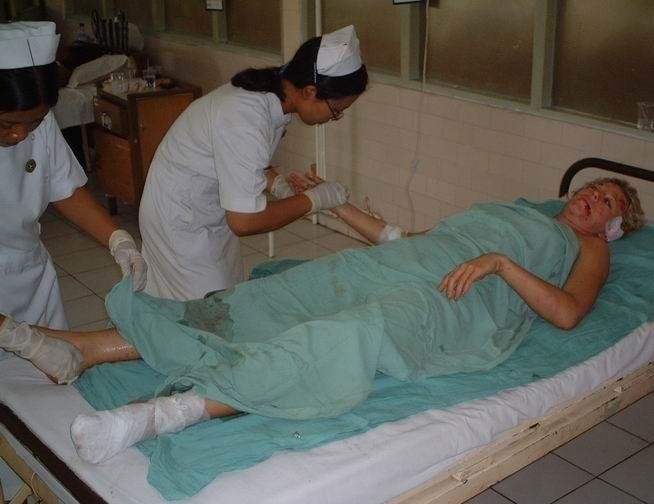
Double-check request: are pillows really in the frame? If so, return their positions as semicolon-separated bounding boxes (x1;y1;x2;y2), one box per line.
65;54;129;88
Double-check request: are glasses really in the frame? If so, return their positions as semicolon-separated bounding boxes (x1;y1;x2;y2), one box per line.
323;97;344;120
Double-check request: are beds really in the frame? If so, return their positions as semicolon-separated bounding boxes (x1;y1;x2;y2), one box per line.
0;155;654;504
51;81;110;174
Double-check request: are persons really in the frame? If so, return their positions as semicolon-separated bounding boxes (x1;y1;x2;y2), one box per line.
0;168;646;466
0;15;147;385
135;20;370;303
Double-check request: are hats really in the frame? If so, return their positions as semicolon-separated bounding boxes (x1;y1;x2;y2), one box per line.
0;18;61;71
314;23;360;80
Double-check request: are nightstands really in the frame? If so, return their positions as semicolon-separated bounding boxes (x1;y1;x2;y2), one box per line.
92;79;203;217
